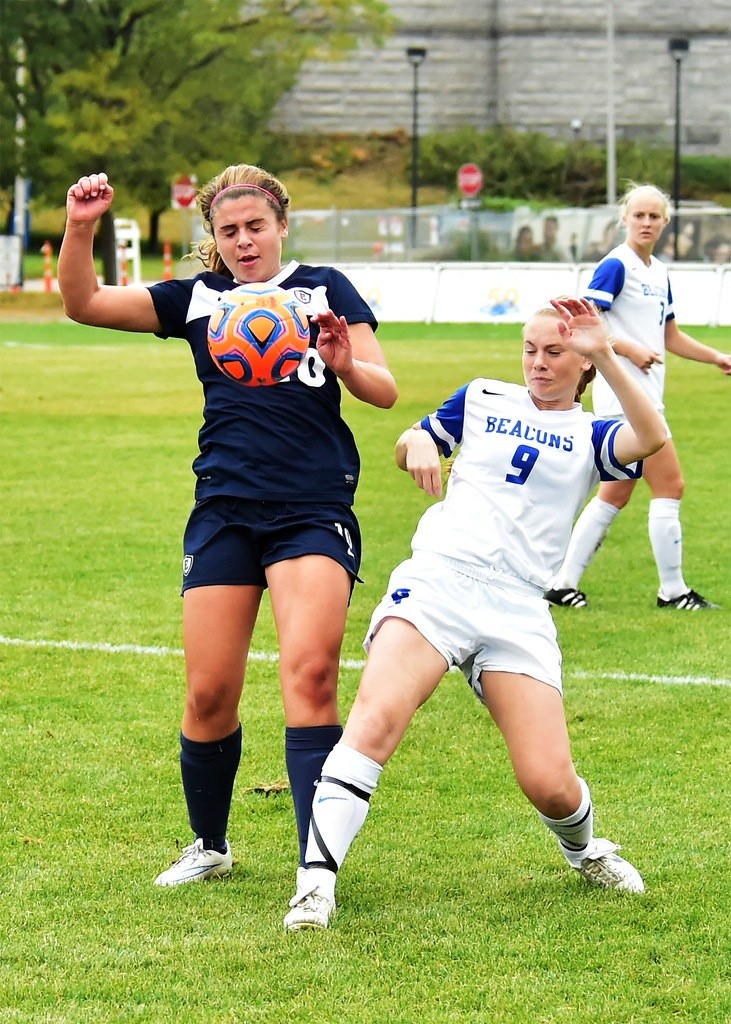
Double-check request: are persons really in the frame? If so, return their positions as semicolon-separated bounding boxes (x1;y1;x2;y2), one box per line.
544;175;731;612
55;162;398;890
283;299;669;936
513;212;731;262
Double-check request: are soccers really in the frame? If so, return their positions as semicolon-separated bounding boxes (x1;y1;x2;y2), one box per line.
208;283;310;386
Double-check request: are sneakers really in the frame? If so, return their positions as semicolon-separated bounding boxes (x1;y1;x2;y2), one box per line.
153;837;232;888
564;836;645;896
544;588;587;611
284;867;336;932
657;589;722;611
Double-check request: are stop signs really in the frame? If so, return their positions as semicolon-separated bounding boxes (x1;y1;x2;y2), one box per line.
172;174;197;204
457;162;484;194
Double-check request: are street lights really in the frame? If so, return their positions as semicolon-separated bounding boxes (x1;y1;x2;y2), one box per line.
405;46;428;251
668;37;690;262
